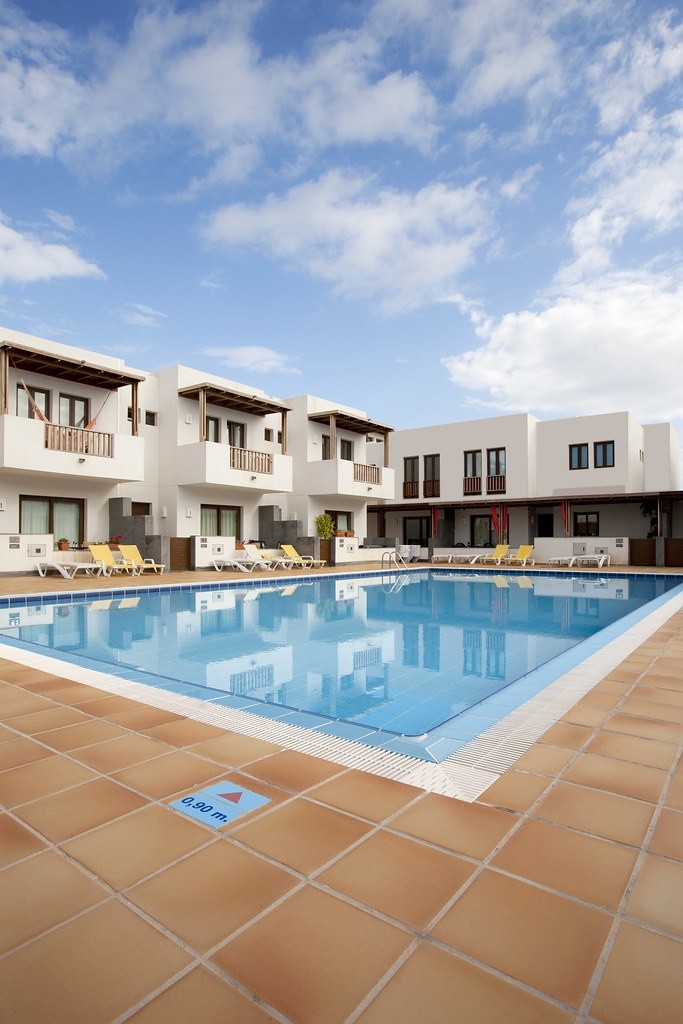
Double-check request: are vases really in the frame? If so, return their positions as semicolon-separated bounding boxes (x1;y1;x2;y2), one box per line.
108;543;122;550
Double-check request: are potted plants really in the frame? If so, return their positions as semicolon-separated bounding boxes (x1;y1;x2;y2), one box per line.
58;538;70;551
337;530;345;537
56;607;70;617
236;541;245;550
345;529;355;537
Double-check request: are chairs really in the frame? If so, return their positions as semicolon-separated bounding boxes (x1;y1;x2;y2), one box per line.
90;545;137;577
117;544;165;575
234;559;272;571
510;576;533;589
37;561;102;581
209;559;251;573
278;584;306;596
281;545;326;569
243;544;273;571
479;545;510;565
453;554;482;564
548;556;578;568
261;552;294;570
486;575;509;589
431;555;455;564
576;554;611;567
506;545;535;566
89;600;113;611
117;598;140;608
244;588;274;602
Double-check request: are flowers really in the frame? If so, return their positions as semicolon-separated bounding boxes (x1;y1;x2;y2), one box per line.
105;534;123;544
